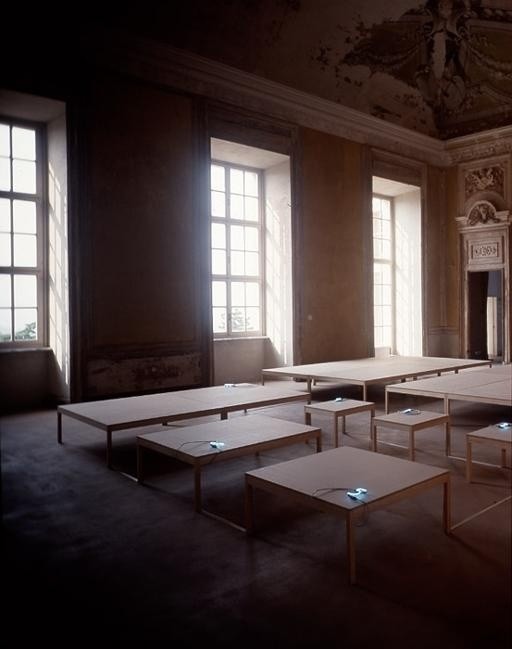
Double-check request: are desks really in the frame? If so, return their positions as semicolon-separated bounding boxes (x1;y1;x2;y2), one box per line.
137;418;321;516
466;423;512;483
262;355;492;401
385;367;511;414
373;411;450;461
305;398;375;446
245;446;450;583
59;386;310;472
